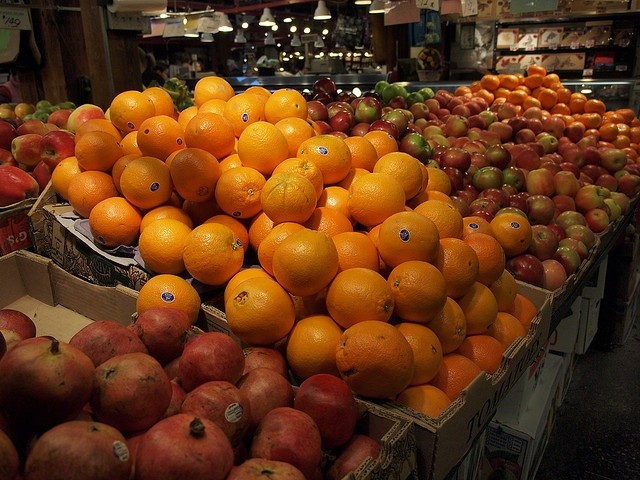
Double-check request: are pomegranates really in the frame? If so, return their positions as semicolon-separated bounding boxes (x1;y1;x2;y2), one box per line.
0;308;378;479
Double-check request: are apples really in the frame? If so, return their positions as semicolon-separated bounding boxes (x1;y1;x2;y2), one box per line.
1;100;106;205
299;79;639;289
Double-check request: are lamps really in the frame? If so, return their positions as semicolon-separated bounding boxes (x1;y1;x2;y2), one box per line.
201;32;214;42
217;14;233;32
242;22;248;28
184;30;199;37
304;25;310;33
322;29;328;34
355;0;372;5
369;0;386;14
259;8;276;26
291;34;300;46
233;28;247;43
272;23;279;31
313;0;331;20
283;16;292;22
263;31;274;45
314;36;325;48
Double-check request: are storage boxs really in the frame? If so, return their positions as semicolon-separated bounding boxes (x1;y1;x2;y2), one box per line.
555;234;600;322
486;353;562;478
1;249;418;480
44;204;553;479
598;296;640;345
603;194;638;249
576;288;604;355
545;297;583;411
0;176;64;259
609;238;640;305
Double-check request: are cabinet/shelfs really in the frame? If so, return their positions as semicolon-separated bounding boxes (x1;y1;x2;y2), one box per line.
493;19;634;77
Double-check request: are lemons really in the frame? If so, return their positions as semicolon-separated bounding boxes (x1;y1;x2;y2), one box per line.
51;77;538;419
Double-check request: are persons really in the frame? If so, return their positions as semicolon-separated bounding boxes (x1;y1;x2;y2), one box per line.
293;58;305;76
157;54;183;65
1;60;36;104
460;48;493;79
194;59;203;72
181;56;193;74
137;46;156;90
226;48;244;72
143;64;170;89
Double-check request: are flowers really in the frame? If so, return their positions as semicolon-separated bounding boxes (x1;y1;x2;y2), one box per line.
418;48;445;69
